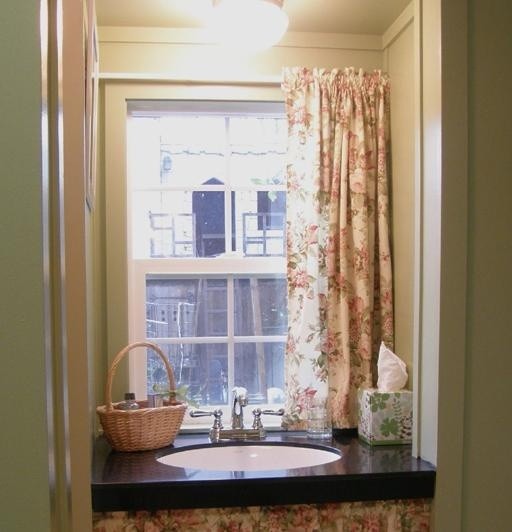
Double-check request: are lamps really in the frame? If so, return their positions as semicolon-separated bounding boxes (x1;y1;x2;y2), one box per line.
212;0;290;49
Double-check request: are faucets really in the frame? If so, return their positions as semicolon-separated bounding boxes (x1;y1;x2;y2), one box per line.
229;384;249;431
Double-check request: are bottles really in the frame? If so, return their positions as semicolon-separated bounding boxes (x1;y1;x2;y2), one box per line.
118;393;140;410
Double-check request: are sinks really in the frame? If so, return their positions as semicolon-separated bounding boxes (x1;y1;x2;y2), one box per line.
154;439;344;474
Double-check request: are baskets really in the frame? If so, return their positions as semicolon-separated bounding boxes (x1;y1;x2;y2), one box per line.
96;342;188;453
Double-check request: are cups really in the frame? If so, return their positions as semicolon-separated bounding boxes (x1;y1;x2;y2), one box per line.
294;395;334;440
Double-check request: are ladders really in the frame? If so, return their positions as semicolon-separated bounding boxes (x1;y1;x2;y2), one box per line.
198;234;243;406
